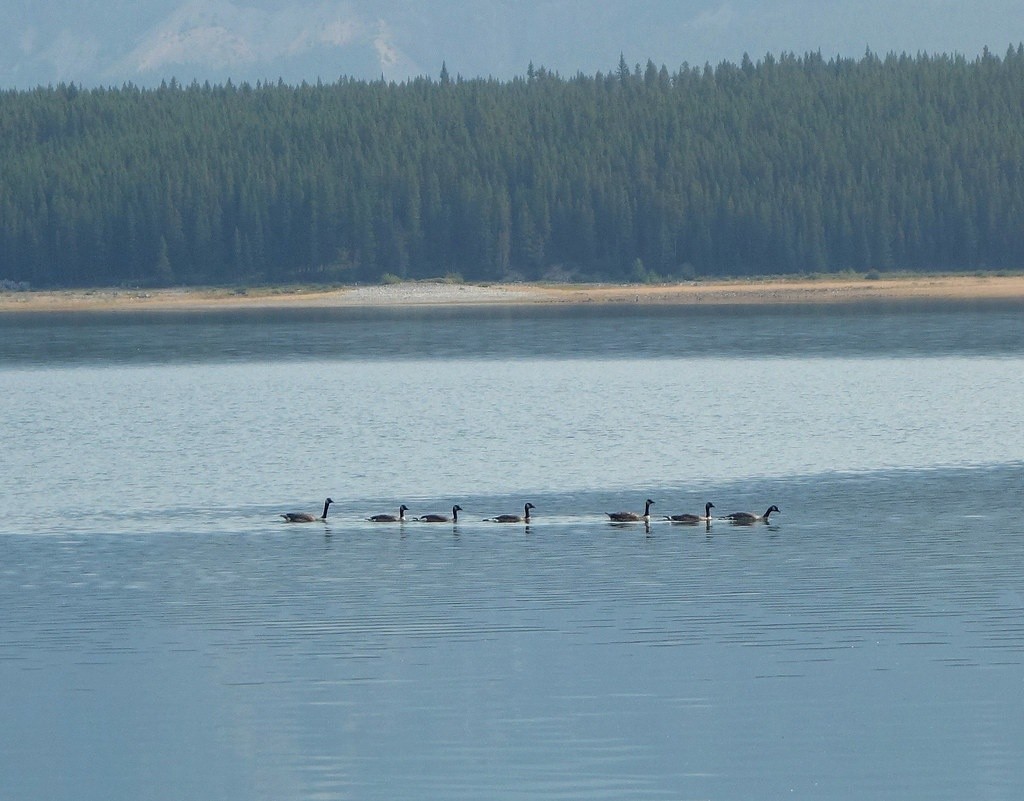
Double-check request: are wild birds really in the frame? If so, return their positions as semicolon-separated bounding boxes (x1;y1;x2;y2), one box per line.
413;505;462;522
482;502;536;523
663;501;716;521
364;505;410;522
605;499;656;522
716;504;780;520
280;498;335;522
